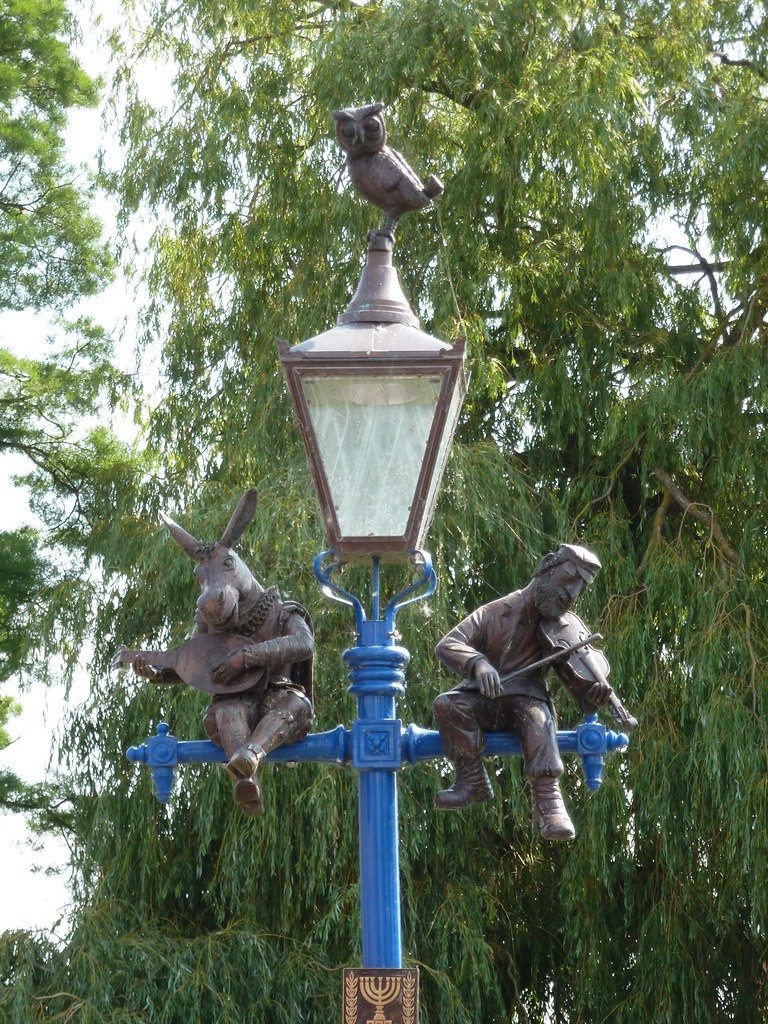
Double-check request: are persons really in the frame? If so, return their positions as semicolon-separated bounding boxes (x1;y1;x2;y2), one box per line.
431;543;614;843
